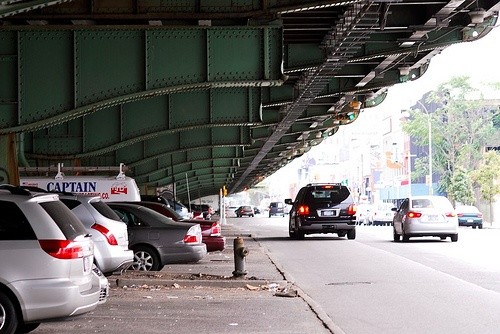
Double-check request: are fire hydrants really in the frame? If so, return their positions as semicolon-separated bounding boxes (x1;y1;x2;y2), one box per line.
232;235;249;279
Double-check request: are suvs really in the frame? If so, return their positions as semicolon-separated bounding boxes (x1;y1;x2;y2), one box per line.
269;182;357;240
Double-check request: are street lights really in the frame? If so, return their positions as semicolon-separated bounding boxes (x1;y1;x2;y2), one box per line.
347;93;435;196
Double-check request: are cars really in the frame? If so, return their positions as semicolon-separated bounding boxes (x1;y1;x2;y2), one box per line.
357;203;396;224
391;195;458;243
103;189;226;274
235;205;262;217
454;205;482;229
0;184;135;334
